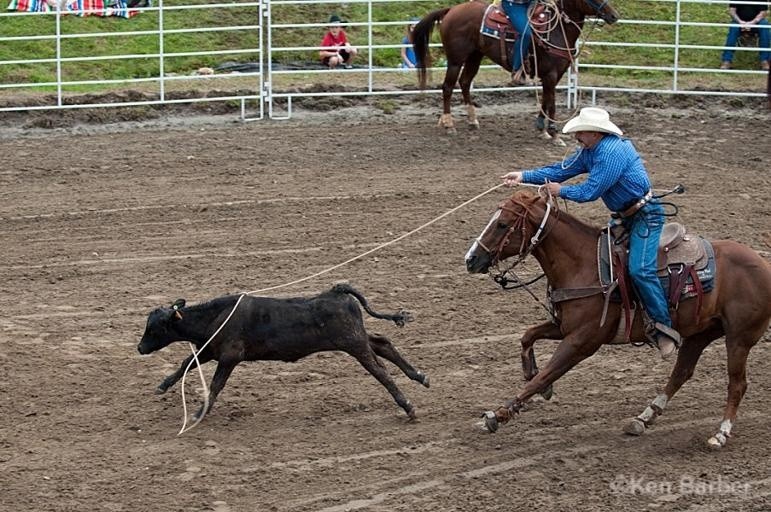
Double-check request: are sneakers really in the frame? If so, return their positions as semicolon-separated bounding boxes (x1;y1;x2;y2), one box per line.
340;62;353;69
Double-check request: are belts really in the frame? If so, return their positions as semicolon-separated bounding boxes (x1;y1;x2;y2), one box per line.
619;188;654;221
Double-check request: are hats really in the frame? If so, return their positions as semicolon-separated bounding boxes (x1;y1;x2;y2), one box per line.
561;106;625;138
321;16;348;28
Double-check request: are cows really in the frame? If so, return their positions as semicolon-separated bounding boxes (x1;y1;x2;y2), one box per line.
136;281;431;423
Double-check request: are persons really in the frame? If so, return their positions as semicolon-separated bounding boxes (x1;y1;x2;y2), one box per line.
718;1;771;71
317;14;354;70
401;17;423;70
502;103;679;358
499;1;542;87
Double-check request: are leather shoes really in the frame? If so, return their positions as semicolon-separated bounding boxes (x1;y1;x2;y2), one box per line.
718;60;735;70
657;335;677;360
511;74;528;86
760;60;771;71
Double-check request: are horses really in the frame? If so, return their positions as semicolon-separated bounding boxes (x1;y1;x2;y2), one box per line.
408;1;621;148
464;189;771;452
